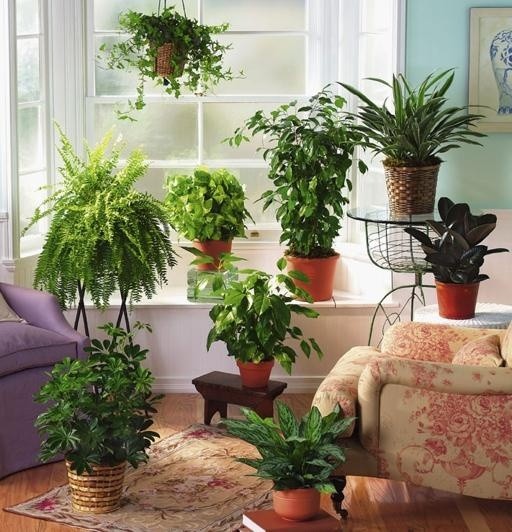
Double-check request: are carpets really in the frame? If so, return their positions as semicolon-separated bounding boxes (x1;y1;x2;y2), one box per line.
3;424;285;532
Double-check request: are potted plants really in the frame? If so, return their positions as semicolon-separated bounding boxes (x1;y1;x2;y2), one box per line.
218;401;357;523
33;321;164;511
223;83;369;303
177;242;324;389
162;169;256;273
402;196;509;319
336;66;498;215
92;5;247;123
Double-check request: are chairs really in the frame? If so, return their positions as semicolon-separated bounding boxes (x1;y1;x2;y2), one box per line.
0;282;93;480
305;313;511;518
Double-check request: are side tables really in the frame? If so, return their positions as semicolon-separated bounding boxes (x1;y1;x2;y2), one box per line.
413;303;512;329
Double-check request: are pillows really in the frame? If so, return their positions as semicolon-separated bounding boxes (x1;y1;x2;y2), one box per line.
0;290;29;324
450;334;505;368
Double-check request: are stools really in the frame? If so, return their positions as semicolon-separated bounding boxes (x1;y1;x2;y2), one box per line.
192;371;286;424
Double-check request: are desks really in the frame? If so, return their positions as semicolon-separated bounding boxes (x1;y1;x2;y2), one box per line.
346;204;482;358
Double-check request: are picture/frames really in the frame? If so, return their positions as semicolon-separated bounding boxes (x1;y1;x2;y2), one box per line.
468;7;512;133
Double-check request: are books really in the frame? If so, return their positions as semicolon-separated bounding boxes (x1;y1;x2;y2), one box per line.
242;505;340;532
